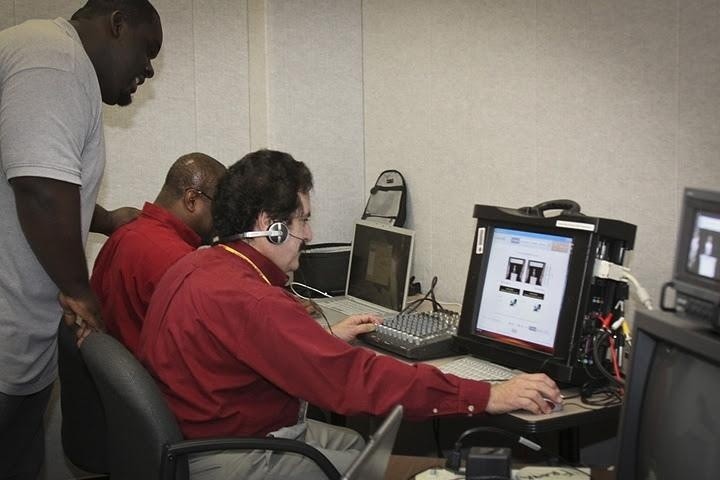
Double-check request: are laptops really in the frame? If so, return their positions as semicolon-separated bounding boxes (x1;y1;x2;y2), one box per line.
313;218;416;332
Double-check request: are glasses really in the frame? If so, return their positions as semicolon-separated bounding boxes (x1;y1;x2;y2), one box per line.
186;188;213;202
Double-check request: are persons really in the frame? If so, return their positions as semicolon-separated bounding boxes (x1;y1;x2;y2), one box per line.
525;268;541;287
0;0;164;480
90;152;322;361
506;264;518;281
136;148;563;480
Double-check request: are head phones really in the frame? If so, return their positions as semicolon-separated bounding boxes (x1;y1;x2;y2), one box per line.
212;221;289;246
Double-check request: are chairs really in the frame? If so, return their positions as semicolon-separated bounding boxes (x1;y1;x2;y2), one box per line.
56;315;111;474
79;330;343;480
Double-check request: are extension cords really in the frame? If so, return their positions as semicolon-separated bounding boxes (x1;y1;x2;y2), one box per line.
414;464;591;480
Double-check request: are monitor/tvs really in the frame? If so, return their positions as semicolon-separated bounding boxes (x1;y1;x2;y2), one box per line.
614;306;720;480
454;203;637;385
659;184;720;322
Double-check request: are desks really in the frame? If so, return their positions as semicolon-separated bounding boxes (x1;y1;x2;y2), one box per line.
301;295;624;466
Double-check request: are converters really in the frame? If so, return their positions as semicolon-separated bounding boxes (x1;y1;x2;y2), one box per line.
464;445;512;480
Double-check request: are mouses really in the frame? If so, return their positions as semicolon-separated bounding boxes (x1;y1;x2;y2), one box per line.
539;397;564;413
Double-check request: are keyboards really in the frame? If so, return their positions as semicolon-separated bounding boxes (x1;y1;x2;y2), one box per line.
440;355;524;384
